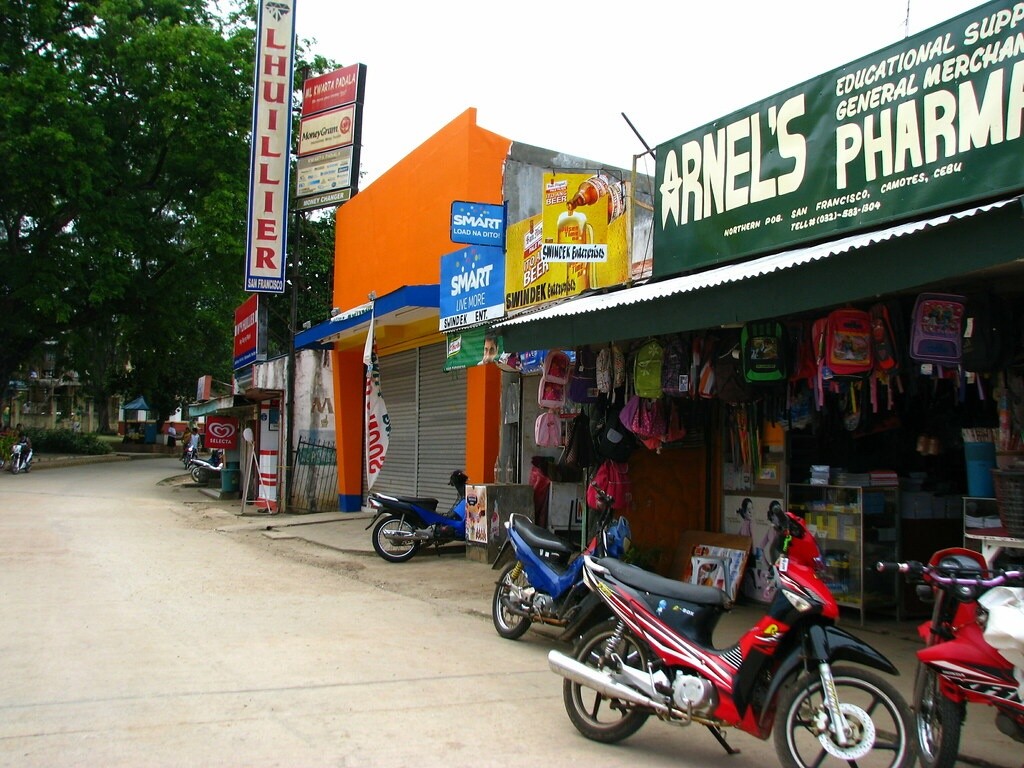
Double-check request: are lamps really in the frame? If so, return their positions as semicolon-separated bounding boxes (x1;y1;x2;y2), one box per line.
368;291;377;300
331;308;340;316
303;321;311;329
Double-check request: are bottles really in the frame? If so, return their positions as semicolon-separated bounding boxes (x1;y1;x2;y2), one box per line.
494;456;502;485
506;456;513;484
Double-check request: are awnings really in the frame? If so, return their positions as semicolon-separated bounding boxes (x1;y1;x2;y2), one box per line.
491;195;1024;355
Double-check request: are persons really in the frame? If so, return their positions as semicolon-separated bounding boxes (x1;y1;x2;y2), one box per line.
167;422;177;454
10;423;31;466
737;498;757;555
761;500;782;570
180;418;201;460
71;410;81;430
478;327;498;365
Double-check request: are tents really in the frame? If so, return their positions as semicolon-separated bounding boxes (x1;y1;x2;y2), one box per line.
123;396;150;422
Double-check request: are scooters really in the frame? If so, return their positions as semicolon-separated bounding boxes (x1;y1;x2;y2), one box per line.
8;443;35;475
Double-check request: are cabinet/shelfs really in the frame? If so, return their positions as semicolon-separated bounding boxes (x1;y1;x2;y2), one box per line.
787;484;898;626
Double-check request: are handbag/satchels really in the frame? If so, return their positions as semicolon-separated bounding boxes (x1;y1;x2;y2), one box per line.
587;459;622;510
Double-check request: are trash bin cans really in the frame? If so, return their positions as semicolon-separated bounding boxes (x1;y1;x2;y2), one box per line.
220;469;240;493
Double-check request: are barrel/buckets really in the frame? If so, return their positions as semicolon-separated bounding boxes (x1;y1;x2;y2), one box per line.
991;469;1024;538
963;442;997;497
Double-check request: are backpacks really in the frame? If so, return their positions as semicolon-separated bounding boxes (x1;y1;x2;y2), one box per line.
556;289;1001;474
538;377;565;409
543;348;570;385
534;410;561;448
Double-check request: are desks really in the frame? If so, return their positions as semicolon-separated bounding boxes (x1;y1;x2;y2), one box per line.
962;533;1023;573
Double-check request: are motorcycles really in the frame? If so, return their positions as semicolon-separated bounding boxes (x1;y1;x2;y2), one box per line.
491;481;638;641
364;469;473;563
179;440;198;471
187;449;225;483
549;503;919;767
874;547;1024;768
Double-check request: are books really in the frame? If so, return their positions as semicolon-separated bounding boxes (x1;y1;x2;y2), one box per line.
833;470;899;487
